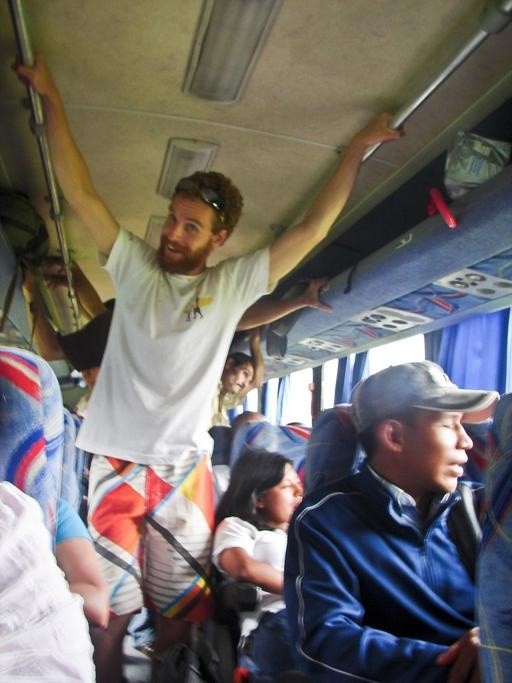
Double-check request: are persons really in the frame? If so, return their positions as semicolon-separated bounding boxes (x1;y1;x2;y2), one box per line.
49;504;112;632
11;49;406;682
278;361;502;682
1;478;102;683
467;393;512;680
23;252;115;372
212;326;267;428
238;275;334;331
210;448;305;674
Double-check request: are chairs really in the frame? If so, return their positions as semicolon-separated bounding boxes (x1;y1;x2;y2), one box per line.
2;346;88;563
200;392;512;683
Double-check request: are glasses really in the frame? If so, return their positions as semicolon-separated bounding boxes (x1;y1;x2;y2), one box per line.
174;178;225;212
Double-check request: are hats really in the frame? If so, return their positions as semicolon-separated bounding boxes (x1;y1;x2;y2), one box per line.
349;362;502;435
